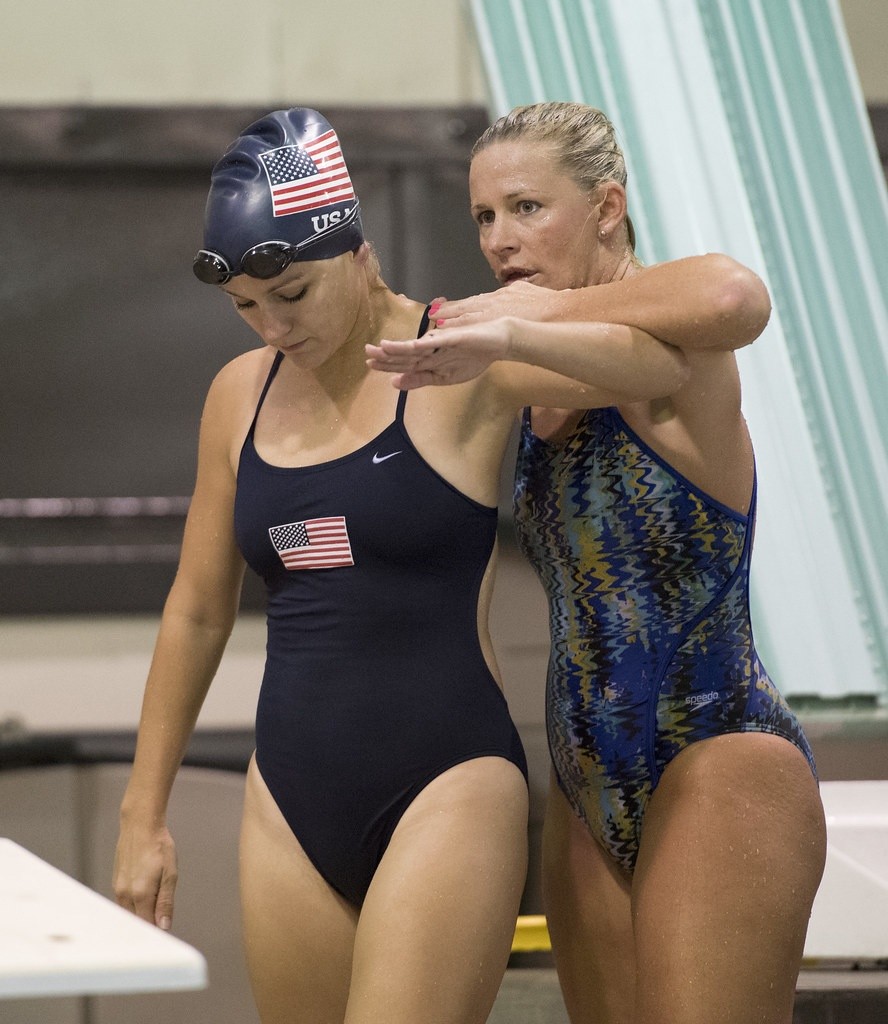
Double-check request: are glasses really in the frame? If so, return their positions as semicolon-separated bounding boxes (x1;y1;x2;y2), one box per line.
192;238;295;285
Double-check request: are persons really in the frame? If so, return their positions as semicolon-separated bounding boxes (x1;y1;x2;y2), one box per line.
114;107;692;1024
431;104;827;1023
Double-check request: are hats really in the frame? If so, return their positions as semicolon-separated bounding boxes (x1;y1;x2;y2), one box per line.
202;107;365;262
